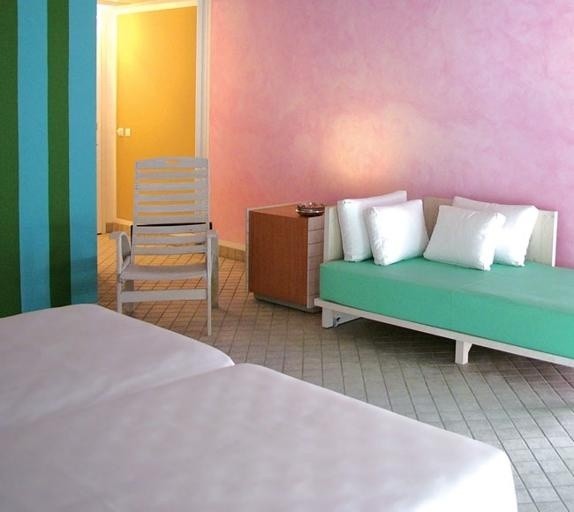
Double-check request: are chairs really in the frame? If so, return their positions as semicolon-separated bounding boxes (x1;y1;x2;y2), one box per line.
115;156;219;336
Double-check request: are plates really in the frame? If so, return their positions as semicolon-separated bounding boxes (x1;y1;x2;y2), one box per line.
297;205;324;216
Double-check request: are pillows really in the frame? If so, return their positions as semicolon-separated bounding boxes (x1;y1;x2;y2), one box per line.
364;199;429;265
423;205;506;272
452;196;539;267
336;190;406;262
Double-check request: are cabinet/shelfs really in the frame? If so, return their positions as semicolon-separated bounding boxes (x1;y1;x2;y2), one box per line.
245;201;325;314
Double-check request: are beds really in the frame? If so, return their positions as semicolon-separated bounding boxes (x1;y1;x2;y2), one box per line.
315;196;574;372
2;361;510;512
0;302;237;427
